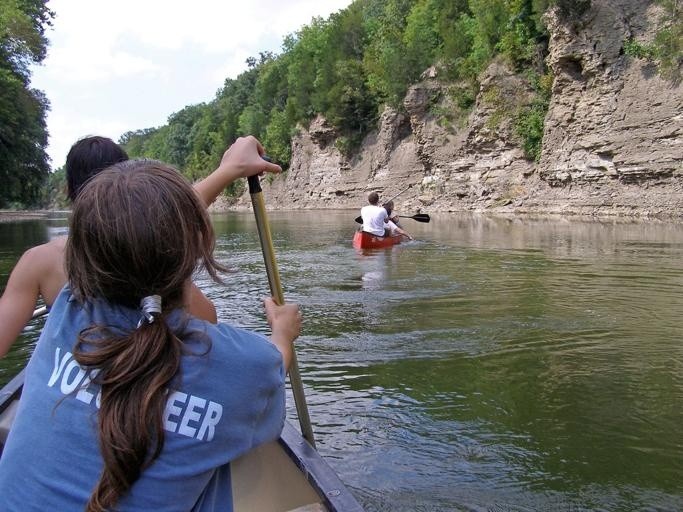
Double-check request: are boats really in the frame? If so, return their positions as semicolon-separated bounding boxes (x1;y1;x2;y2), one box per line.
351;229;402;250
1;360;366;512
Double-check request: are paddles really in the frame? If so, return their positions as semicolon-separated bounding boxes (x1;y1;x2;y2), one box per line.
400;214;429;223
355;184;412;224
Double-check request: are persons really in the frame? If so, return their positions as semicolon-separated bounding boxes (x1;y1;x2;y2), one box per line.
388;201;402;228
360;191;389;238
1;135;304;512
0;136;219;359
415;207;421;215
381;204;413;241
